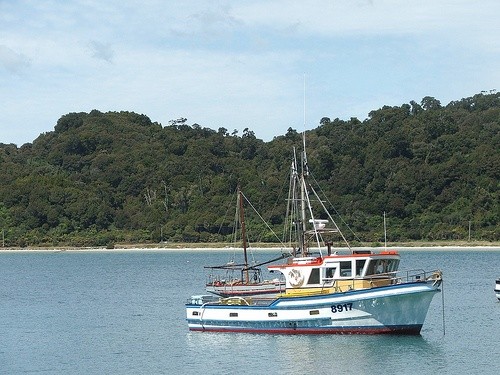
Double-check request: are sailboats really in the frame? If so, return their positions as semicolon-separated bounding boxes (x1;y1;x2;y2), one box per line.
184;146;442;336
